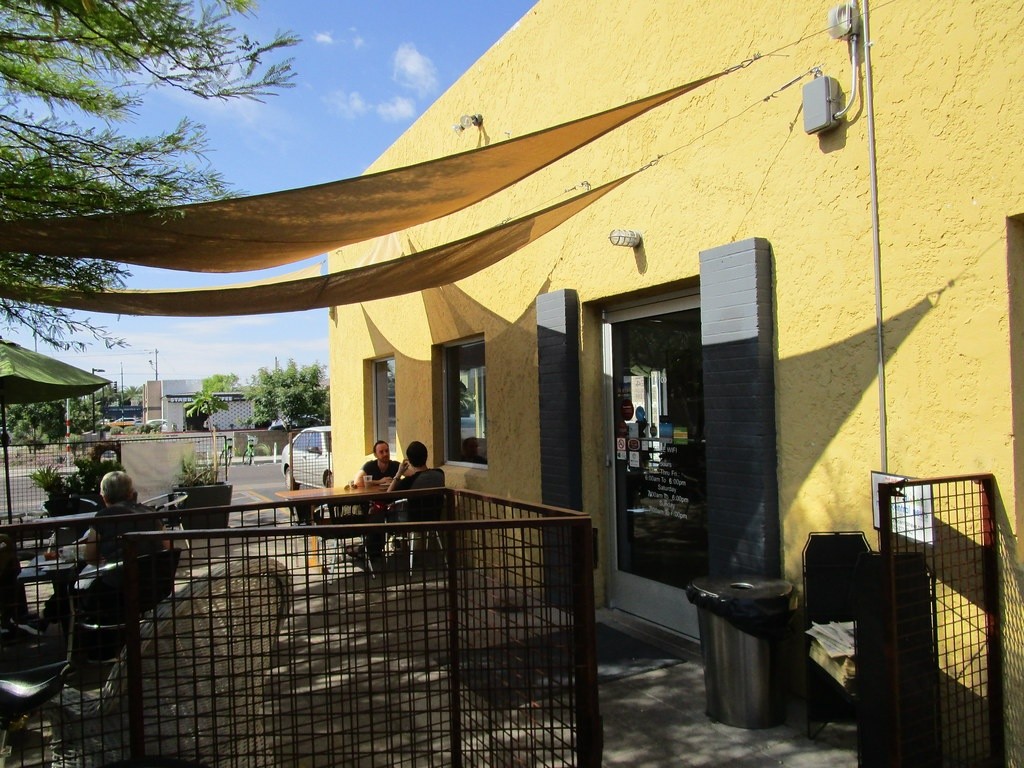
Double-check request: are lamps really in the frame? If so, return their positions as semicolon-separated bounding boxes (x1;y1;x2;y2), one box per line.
608;229;640;247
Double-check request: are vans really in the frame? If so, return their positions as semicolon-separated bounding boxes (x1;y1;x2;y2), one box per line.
100;414;144;427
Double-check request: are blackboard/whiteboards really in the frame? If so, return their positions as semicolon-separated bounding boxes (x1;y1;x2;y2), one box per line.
869;471;936;547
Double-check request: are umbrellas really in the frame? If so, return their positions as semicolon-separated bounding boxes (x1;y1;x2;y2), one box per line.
0;335;114;525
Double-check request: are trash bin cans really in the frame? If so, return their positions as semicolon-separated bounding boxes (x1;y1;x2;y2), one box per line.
691;572;793;730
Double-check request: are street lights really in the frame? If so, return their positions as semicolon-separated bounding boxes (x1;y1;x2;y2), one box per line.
120;361;124;422
92;368;105;433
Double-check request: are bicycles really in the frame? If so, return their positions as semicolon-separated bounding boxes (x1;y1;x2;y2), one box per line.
242;433;257;466
219;433;232;467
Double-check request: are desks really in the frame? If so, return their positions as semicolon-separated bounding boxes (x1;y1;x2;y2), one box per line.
272;484;444;575
16;548;90;583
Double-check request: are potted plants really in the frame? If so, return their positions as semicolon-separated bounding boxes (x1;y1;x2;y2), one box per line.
171;445;232;538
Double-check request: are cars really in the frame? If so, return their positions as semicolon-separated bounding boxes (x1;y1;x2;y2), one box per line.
281;426;333;490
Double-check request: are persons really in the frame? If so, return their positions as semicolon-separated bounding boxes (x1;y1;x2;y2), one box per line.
633;407;651;469
459;436;487;465
356;440;447;558
8;471;172;636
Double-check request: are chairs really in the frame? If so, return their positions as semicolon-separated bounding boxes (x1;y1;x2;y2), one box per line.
313;498;379;580
141;491;195;557
48;548;295;768
384;494;449;575
